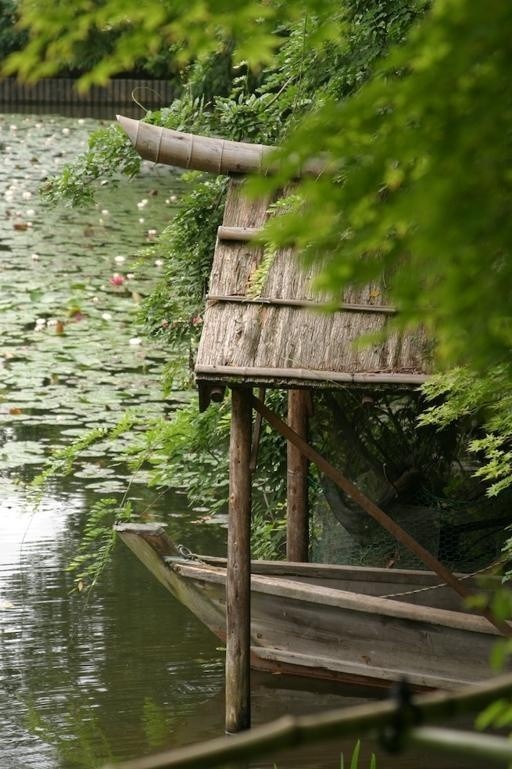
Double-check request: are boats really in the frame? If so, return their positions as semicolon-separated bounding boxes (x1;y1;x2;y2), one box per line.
109;514;512;704
